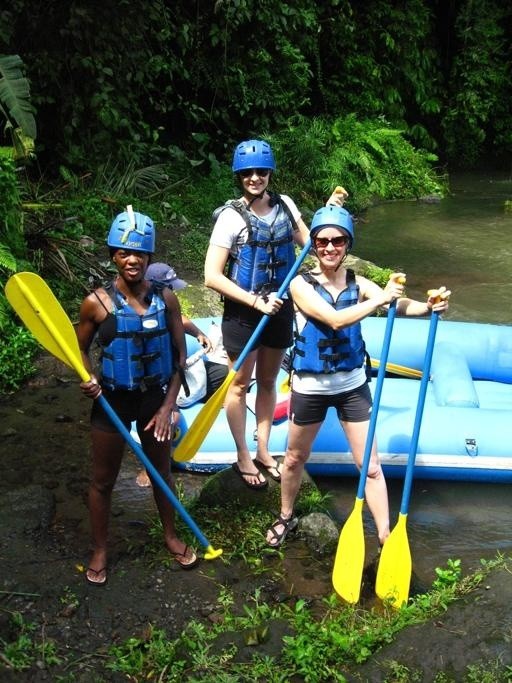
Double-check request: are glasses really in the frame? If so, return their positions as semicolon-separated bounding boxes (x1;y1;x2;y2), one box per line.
315;235;347;248
240;168;268;177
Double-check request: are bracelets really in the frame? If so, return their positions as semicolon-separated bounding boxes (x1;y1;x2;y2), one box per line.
252;295;259;307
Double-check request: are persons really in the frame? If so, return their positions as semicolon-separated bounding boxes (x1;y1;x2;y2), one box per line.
204;140;344;490
77;204;198;586
264;206;451;549
135;262;228;488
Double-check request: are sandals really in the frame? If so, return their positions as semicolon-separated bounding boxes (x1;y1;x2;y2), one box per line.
266;514;298;547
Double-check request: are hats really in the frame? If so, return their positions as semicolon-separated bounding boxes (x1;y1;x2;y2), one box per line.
144;262;187;290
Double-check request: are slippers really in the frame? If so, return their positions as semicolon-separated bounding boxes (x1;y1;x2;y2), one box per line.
164;542;199;568
255;459;282;480
86;567;108;586
232;463;268;488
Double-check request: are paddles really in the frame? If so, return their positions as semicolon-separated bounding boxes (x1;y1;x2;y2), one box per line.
374;289;443;608
370;358;423;380
332;273;407;606
4;271;222;559
172;186;346;464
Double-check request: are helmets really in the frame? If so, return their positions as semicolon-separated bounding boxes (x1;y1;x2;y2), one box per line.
108;211;155;253
232;140;274;174
310;206;354;242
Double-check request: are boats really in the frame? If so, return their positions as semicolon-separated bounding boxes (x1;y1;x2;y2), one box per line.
125;310;512;481
